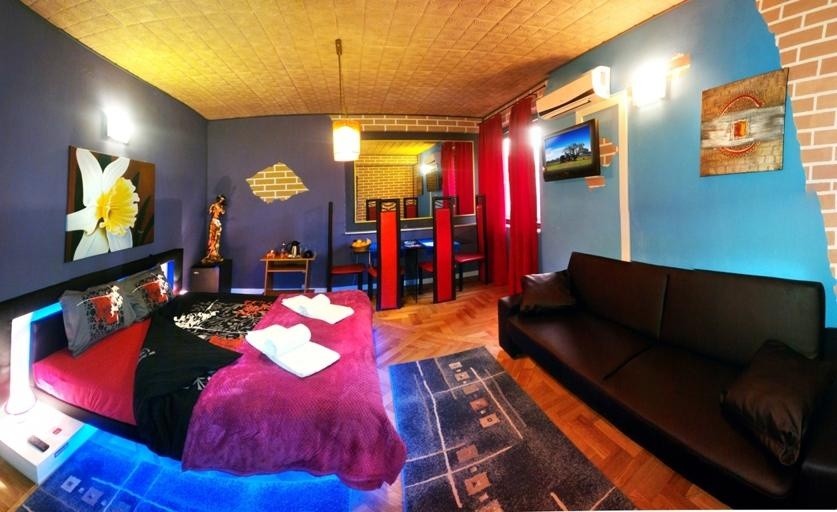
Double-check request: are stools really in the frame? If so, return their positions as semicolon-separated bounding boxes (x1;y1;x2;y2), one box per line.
352;247;367;284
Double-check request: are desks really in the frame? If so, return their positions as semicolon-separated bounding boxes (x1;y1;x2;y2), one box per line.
259;252;317;295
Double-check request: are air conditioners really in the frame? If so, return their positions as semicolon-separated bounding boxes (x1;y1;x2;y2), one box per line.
536;66;610;121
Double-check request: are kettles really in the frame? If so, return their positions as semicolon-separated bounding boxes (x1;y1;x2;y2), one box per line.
286;240;301;257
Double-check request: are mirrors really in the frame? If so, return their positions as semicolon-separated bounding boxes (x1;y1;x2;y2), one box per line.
353;137;475;224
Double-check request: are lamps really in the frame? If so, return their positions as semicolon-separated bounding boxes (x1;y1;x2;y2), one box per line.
332;38;361;161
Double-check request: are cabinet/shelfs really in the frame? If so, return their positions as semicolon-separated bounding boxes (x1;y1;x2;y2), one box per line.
191;258;233;293
1;248;407;485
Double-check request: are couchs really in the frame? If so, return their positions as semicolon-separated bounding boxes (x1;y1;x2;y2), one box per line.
498;252;837;509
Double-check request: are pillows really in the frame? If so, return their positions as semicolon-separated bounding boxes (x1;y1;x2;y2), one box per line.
118;263;175;323
717;338;814;466
60;282;136;357
520;269;575;315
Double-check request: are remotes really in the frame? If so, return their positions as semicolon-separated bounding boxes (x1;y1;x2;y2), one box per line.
28;434;50;452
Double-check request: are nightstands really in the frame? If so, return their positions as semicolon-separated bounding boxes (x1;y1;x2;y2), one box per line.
0;401;83;485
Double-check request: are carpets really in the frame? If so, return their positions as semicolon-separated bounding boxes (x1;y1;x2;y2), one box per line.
14;429;350;512
388;345;640;512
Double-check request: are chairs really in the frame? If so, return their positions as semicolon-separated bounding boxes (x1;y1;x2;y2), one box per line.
418;195;456;303
454;194;489;292
403;197;418;218
368;198;406;311
366;199;381;221
326;201;366;292
